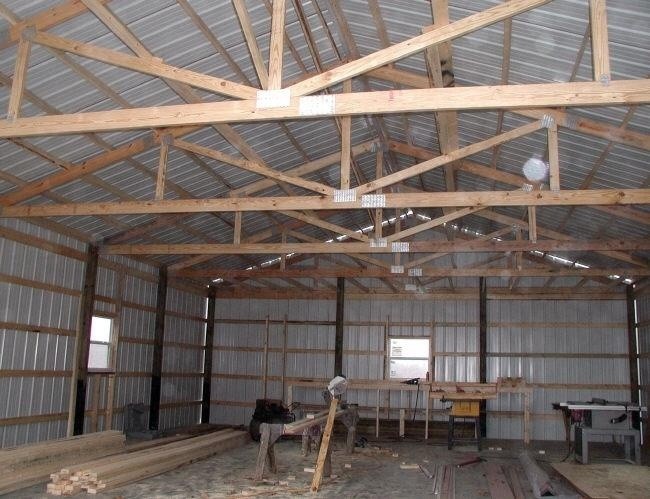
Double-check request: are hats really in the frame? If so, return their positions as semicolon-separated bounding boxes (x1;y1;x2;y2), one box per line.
328;376;348;396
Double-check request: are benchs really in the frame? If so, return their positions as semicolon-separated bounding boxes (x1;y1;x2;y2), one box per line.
574;425;640;465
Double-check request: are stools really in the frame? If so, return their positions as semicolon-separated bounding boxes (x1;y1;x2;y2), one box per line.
448;400;483;451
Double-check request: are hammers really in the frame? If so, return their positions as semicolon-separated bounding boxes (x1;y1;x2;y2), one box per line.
457;456;487;467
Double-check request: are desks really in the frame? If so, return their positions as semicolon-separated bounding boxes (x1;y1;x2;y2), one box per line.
556;401;647;467
